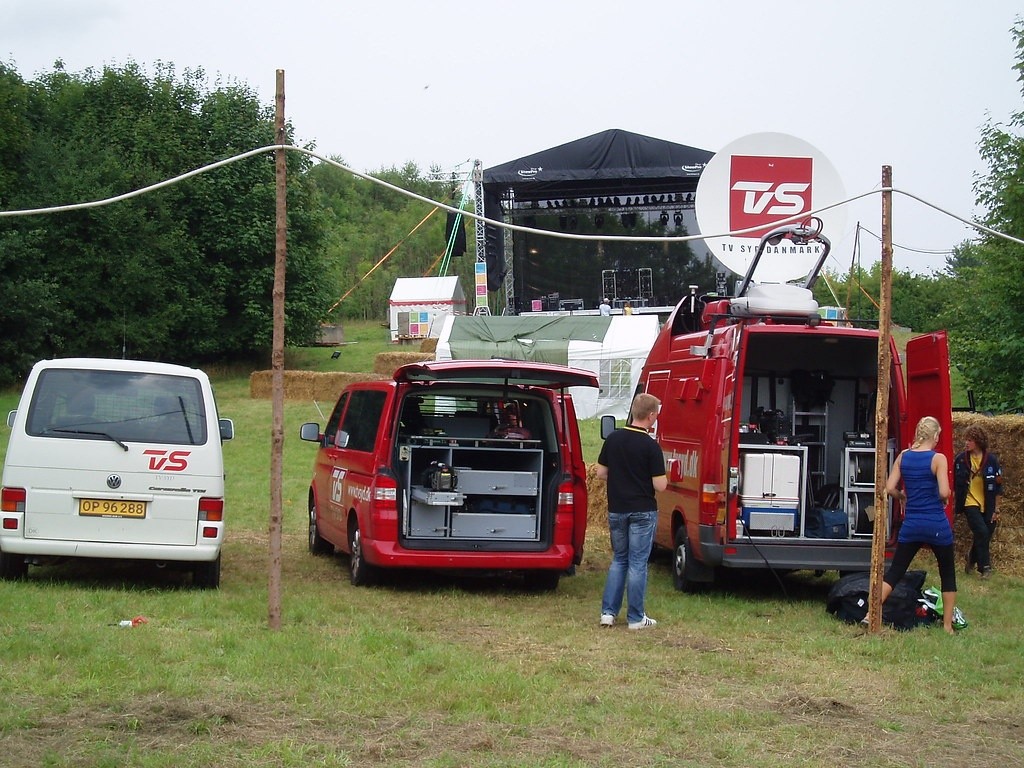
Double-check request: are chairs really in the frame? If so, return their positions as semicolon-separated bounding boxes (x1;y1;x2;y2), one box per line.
56;388;105;435
143;397;196;443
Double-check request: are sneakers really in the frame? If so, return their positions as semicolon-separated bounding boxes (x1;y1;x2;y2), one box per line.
628;612;657;629
600;613;616;626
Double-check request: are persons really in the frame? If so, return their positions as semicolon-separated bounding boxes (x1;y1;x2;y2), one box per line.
953;426;1003;579
599;298;610;316
861;418;960;636
597;394;667;629
623;304;631;315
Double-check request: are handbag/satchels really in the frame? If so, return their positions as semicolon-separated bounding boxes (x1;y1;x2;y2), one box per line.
918;585;968;629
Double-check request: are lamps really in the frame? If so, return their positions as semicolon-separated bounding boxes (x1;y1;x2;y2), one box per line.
621;211;637;229
569;215;578;231
559;214;567;231
674;209;683;226
659;209;669;226
546;192;696;208
594;214;604;229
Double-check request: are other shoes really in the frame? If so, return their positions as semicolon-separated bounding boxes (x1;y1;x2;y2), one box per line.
965;560;977;576
982;566;993;581
860;612;870;627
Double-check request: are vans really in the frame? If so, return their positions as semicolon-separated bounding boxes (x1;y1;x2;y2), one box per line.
600;223;956;589
0;358;236;589
300;357;604;588
